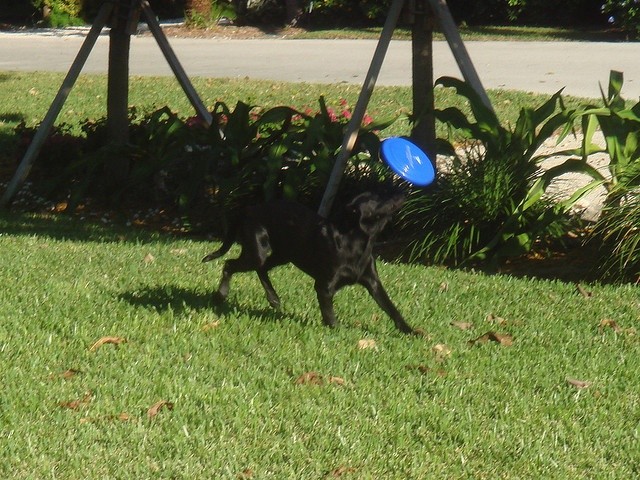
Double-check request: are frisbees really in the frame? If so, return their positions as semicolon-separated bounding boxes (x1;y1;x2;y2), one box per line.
380;137;435;188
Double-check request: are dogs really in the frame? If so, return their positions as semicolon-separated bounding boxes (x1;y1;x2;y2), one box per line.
203;188;416;334
